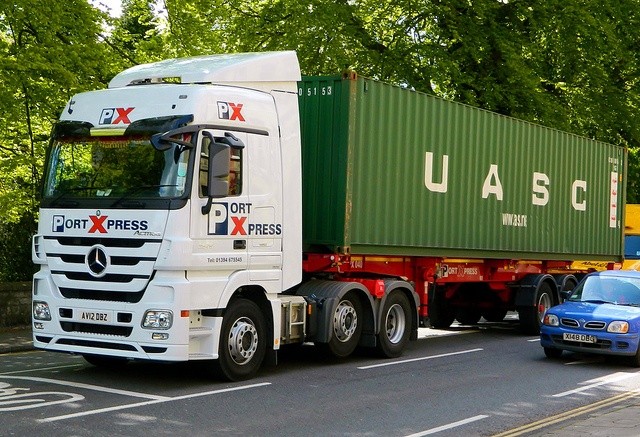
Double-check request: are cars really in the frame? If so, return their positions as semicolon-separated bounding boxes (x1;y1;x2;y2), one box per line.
541;269;640;364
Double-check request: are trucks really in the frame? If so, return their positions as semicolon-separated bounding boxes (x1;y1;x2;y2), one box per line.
30;50;628;379
569;202;640;272
